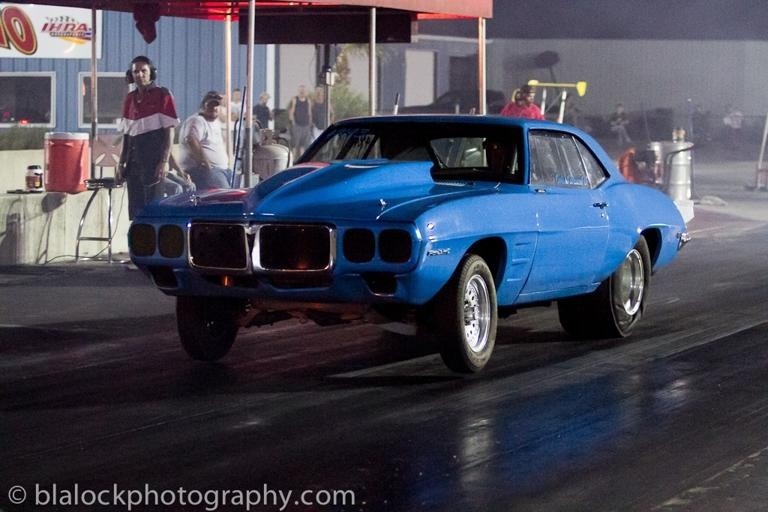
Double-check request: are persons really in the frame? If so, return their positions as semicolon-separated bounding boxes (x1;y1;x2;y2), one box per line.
114;56;183;270
499;84;546;122
177;90;247;191
608;102;633;144
163;153;198;197
217;82;335;167
691;101;745;161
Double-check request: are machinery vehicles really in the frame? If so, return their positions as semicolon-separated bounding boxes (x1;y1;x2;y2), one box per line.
124;111;690;374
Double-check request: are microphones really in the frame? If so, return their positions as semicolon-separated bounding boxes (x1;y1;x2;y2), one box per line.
73;177;123;265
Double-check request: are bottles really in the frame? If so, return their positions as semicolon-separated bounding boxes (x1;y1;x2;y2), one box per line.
202;91;223;108
520;85;536;93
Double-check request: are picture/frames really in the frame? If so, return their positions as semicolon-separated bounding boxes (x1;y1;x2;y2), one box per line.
0;170;262;268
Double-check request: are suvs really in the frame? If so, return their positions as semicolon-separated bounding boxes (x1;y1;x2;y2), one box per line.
648;140;694;202
44;132;89;194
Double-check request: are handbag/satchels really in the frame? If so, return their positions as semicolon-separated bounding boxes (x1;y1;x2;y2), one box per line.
160;159;169;163
119;160;124;167
